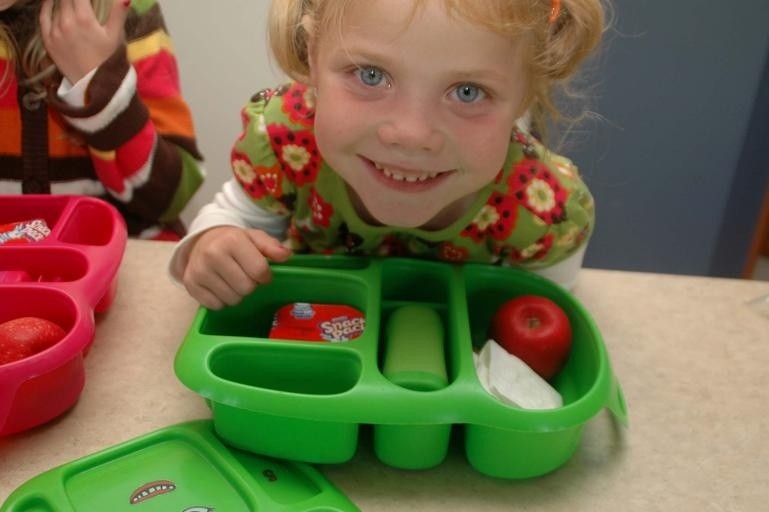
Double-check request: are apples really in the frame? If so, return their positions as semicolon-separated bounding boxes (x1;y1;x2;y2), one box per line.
485;295;573;381
0;317;66;364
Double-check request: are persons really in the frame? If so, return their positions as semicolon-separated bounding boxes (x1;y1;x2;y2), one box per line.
159;0;596;311
0;0;211;246
